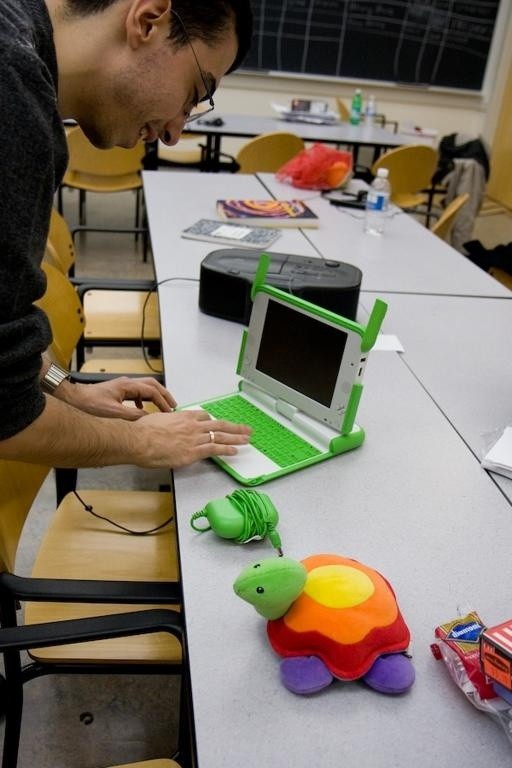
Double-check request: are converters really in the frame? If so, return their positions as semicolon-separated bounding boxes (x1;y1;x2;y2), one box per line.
206;492;279;539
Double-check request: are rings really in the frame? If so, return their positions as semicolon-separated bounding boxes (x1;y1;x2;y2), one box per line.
208;431;218;441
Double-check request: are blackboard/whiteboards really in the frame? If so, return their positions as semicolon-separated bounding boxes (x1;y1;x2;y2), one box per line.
230;1;511;99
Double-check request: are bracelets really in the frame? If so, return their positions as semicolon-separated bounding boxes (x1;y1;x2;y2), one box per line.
40;360;74;396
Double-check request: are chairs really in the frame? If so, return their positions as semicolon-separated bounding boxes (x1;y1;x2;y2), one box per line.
231;131;474;244
0;106;187;763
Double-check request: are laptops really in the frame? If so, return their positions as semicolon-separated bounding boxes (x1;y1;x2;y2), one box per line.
171;252;388;487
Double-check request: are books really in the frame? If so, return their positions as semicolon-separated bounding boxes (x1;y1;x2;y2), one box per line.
181;218;284;250
216;199;320;228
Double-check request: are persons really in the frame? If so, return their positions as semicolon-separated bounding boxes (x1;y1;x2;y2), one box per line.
2;0;255;469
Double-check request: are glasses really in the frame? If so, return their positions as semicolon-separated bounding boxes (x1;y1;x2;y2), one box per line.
169;7;215;124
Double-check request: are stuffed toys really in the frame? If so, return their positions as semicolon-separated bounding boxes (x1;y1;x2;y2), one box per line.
232;552;417;696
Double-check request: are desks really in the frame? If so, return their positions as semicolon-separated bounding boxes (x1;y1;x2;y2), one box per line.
61;108;464;179
136;162;512;766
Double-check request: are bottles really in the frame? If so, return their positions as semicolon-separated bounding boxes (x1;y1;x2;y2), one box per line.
351;89;363;126
365;95;377;129
363;168;392;238
